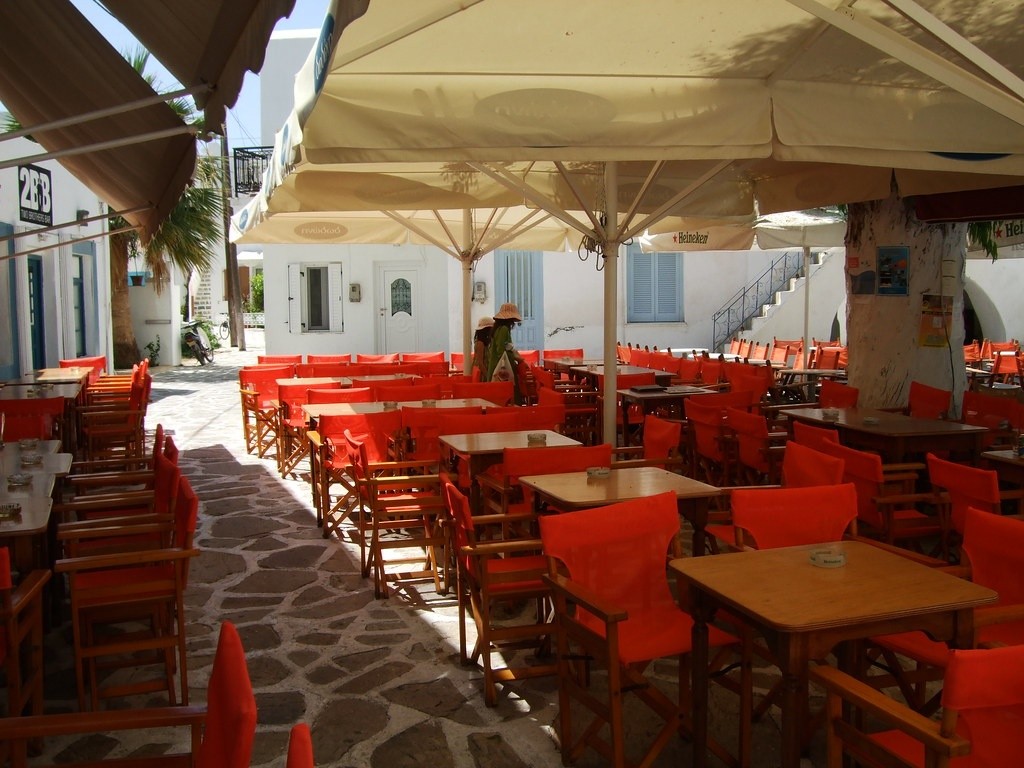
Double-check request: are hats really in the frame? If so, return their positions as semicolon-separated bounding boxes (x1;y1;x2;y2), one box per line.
492;303;523;321
475;316;495;330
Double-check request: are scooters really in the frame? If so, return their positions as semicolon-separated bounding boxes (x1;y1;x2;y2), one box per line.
180;320;214;366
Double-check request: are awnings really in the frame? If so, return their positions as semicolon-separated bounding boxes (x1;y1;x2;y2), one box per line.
0;0;298;263
1;0;294;168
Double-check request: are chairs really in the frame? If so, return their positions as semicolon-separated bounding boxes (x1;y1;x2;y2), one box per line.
0;339;1024;768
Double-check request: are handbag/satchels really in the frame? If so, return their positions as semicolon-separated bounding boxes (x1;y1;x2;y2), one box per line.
491;351;516;386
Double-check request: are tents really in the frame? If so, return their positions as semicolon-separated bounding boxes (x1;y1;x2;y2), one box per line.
228;2;1024;508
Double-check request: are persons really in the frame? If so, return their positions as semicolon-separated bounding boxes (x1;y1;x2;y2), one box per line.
473;302;529;408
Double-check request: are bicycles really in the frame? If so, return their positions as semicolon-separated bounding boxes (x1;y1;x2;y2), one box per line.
219;312;229;340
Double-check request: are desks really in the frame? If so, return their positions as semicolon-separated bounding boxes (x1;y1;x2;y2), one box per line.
520;466;725;691
0;383;83;453
835;419;991;473
0;453;73;477
0;473;57;569
542;358;625;397
759;365;793;369
299;402;403;527
275;377;352;389
729;360;786;366
58;356;108;376
669;540;1001;768
569;366;679;445
779;407;909;430
688;353;741;360
780;368;846;401
399;398;502;476
348;374;423;381
981;448;1024;465
25;366;96;401
0;497;53;577
616;385;721;461
439;431;584;539
659;348;710;353
